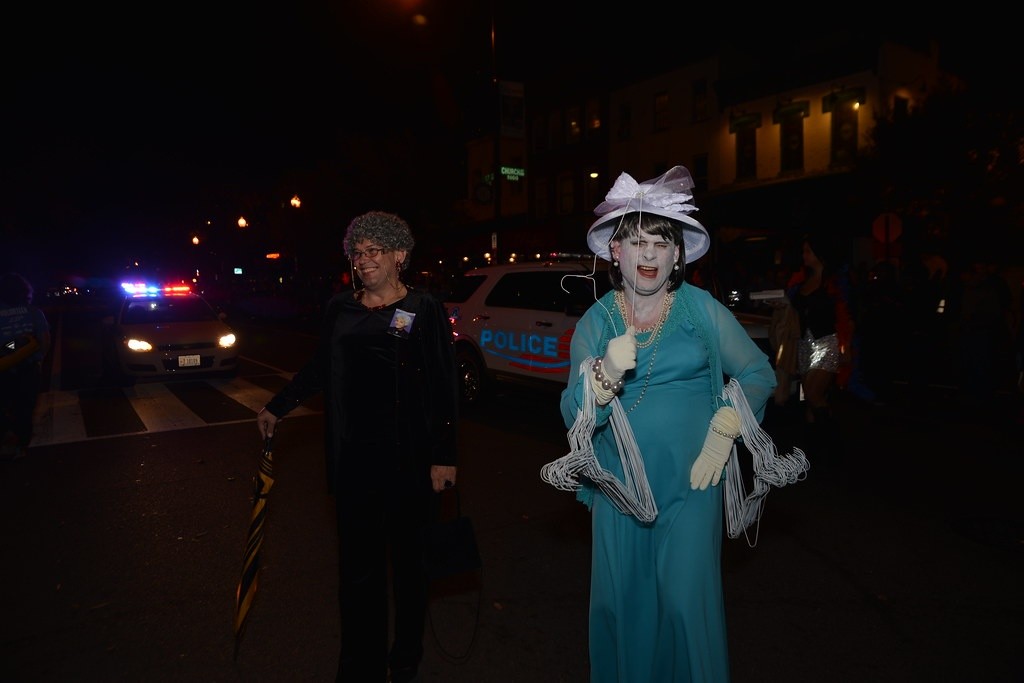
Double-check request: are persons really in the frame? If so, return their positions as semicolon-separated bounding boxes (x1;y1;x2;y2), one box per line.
391;314;410;337
559;165;777;683
257;211;460;683
783;234;840;425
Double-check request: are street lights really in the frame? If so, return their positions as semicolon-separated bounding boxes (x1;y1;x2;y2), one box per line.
413;0;505;266
192;193;302;302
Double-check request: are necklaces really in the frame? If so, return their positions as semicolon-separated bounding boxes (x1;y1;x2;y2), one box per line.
355;288;387;311
601;295;665;415
615;290;671;348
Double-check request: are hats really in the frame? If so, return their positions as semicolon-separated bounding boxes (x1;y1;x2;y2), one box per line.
585;166;710;265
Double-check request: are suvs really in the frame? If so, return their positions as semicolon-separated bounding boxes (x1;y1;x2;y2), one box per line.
442;260;775;406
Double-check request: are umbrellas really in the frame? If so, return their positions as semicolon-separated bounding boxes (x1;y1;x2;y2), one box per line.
231;420;285;664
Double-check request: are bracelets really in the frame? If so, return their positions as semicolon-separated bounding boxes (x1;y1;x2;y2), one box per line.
714;427;739;440
592;357;625;392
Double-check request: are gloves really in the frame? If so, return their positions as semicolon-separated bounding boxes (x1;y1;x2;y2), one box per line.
688;406;742;490
588;326;638;406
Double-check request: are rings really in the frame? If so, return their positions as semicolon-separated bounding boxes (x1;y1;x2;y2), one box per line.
443;479;452;488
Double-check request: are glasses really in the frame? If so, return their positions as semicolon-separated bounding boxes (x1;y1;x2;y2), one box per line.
350;247;388;260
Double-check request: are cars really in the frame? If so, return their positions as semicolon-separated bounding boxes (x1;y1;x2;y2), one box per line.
101;278;241;381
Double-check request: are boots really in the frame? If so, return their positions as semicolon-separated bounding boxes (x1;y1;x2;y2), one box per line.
812;405;839;469
801;423;817;470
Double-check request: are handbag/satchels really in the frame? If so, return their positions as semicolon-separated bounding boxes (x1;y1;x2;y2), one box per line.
430;486;483;572
